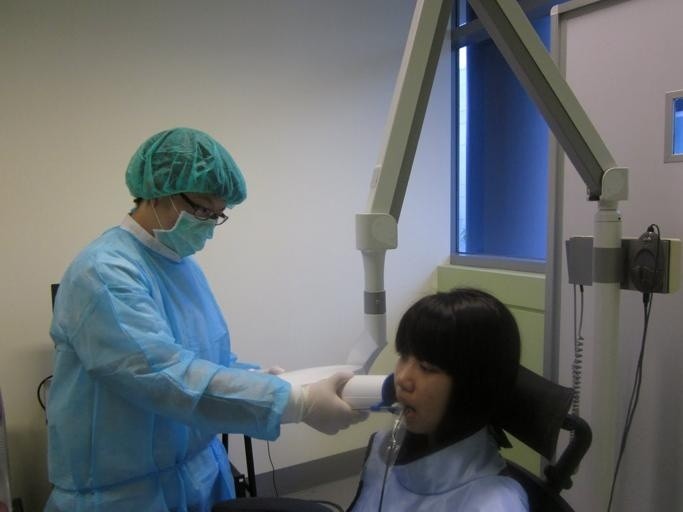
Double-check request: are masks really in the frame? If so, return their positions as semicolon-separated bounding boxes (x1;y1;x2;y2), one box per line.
152;196;216;259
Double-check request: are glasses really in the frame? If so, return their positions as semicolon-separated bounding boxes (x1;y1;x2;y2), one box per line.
179;193;228;224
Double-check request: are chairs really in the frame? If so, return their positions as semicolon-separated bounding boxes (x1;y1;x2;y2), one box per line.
214;358;590;511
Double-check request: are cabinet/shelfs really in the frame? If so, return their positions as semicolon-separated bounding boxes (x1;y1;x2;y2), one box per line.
49;283;257;500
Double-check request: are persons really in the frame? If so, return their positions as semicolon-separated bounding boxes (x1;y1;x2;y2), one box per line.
343;286;530;511
41;126;370;512
